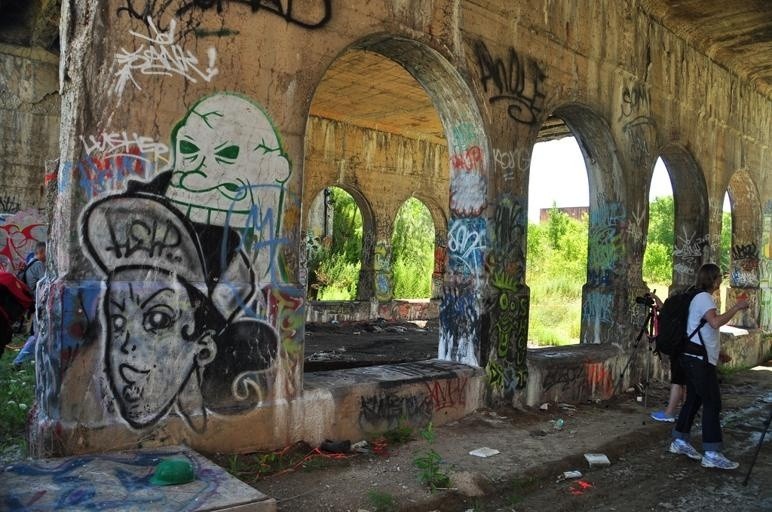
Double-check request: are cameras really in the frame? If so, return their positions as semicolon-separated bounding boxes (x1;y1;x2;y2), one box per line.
636;296;654;306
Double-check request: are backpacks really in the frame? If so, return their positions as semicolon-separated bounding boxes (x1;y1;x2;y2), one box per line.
655;285;713;357
17;259;42;291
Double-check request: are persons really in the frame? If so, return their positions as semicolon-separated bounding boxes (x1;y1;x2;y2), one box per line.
670;263;750;470
0;241;45;374
78;192;279;435
646;284;686;424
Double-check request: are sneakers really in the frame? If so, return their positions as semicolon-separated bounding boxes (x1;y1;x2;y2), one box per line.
700;450;739;470
668;438;704;460
651;409;675;423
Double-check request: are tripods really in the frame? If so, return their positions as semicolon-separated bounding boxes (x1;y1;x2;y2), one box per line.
611;308;658;409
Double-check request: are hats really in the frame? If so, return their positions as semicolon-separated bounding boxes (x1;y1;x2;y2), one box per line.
81;168;256;324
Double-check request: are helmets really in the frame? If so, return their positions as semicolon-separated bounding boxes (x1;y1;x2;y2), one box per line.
149;457;196;487
